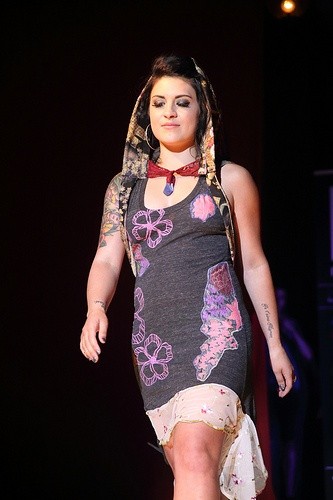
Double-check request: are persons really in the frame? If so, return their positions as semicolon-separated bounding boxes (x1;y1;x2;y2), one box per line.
78;53;296;500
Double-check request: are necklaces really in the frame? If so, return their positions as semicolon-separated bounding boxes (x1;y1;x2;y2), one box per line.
146;160;199;196
